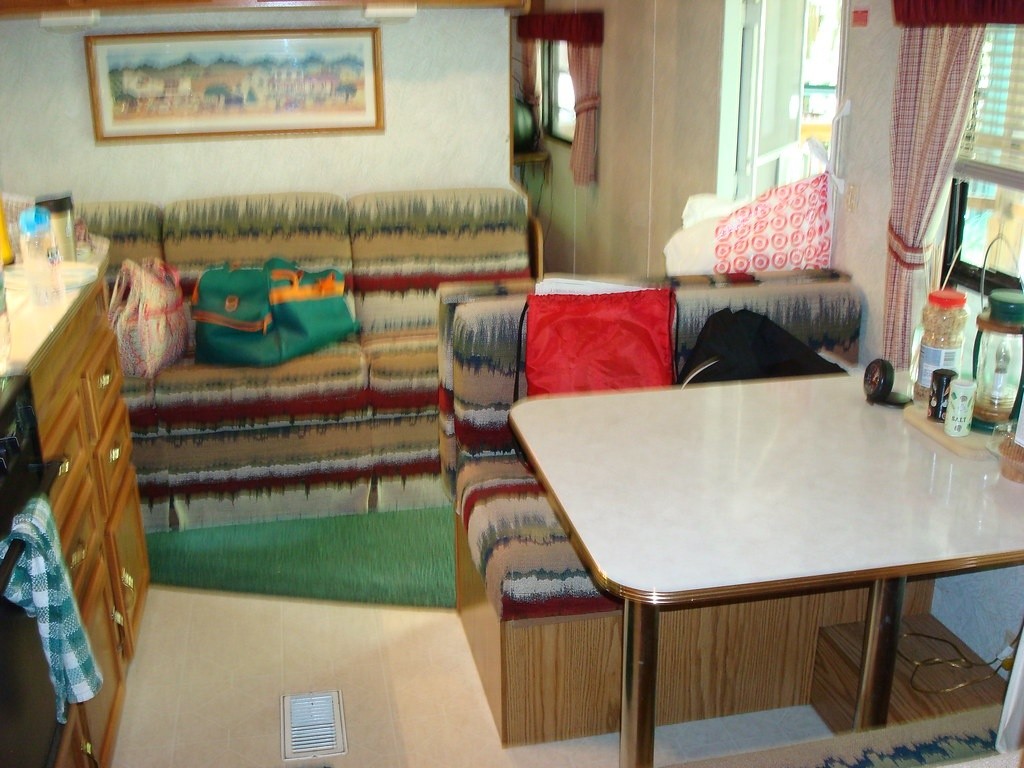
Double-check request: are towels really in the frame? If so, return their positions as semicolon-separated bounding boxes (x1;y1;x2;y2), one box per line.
0;494;103;724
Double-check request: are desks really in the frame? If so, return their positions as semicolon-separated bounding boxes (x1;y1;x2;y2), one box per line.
508;367;1024;768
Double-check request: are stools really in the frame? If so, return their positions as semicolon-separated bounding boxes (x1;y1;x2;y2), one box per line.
513;152;549;188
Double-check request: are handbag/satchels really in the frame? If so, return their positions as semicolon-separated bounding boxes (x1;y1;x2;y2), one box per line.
109;254;190;379
509;275;681;475
683;309;848;385
189;257;363;366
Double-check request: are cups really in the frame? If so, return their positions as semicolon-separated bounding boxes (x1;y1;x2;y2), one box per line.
942;380;978;439
925;367;955;425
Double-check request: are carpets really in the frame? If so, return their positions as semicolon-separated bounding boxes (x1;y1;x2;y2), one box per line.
142;503;462;612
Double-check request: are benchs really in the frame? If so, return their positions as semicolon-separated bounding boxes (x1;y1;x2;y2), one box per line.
430;267;938;751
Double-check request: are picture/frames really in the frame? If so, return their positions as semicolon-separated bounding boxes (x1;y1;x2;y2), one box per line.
84;25;387;145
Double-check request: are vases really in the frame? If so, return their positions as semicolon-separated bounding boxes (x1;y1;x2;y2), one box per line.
512;91;539;155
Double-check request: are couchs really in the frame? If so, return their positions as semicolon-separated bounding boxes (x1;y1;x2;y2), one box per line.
68;186;531;537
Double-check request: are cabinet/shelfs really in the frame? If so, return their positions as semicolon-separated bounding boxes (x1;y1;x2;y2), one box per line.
0;230;148;768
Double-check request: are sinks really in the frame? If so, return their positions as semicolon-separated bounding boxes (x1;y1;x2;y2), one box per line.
0;272;32;321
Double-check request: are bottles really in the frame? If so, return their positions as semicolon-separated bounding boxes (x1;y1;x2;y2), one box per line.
0;192;15;267
914;287;1024;437
18;192;78;309
0;258;11;365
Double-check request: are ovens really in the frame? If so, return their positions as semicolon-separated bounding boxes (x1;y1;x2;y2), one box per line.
0;373;71;768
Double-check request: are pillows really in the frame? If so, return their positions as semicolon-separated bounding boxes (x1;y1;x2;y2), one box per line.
522;287;680;400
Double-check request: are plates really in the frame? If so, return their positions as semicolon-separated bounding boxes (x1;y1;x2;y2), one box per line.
48;261;98;288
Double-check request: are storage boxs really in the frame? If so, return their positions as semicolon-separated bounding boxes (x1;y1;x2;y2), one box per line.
810;612;1008;735
